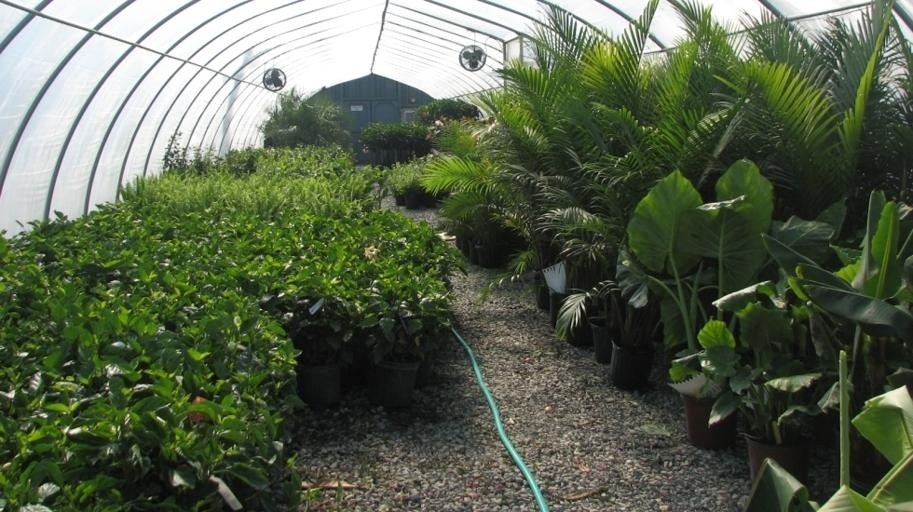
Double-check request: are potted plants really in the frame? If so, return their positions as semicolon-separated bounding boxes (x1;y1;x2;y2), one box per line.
608;158;913;512
0;92;467;410
448;2;913;366
